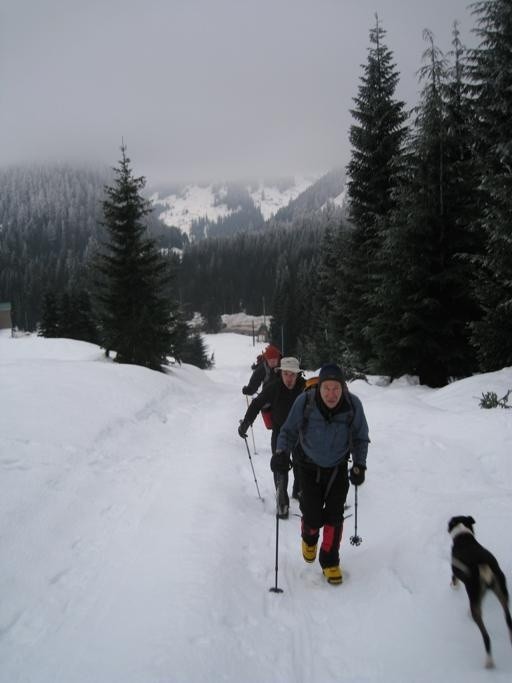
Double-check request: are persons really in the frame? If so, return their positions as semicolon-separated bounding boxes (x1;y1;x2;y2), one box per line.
269;364;370;585
243;343;284;430
238;356;311;520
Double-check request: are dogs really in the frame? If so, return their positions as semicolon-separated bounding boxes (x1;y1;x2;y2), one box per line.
446;514;512;668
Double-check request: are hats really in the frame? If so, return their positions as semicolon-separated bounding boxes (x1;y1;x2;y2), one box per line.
262;344;282;360
317;362;346;392
272;355;304;374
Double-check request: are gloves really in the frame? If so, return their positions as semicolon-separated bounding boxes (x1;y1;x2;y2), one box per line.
349;462;368;487
242;385;254;396
269;450;294;475
237;419;251;439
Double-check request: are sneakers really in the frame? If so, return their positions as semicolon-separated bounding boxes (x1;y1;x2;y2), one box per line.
277;500;290;521
322;565;345;586
301;538;318;564
